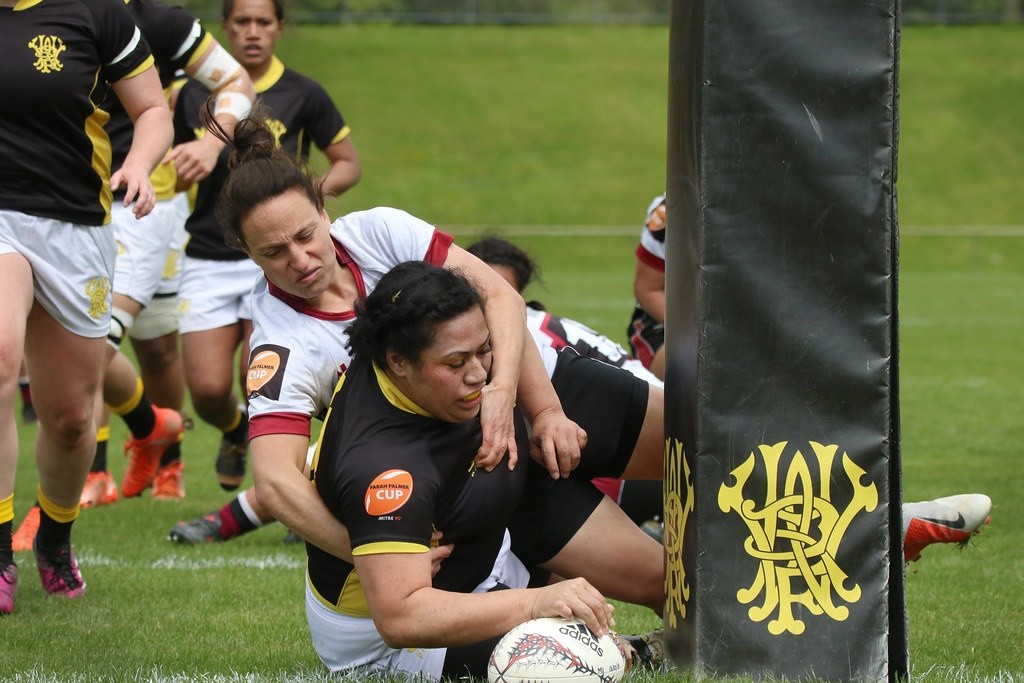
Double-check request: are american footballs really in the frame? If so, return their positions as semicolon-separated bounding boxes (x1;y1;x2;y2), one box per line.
486;616;625;683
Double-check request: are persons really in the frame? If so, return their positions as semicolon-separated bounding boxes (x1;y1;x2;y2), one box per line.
302;261;669;683
0;0;994;616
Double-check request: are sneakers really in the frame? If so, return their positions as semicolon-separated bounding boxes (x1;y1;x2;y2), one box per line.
79;469;118;509
902;494;994;567
121;405;194;497
620;625;665;670
152;460;187;499
32;536;86;598
170;510;222;544
283;528;301;543
11;507;41;551
0;556;18;615
216;404;251;490
